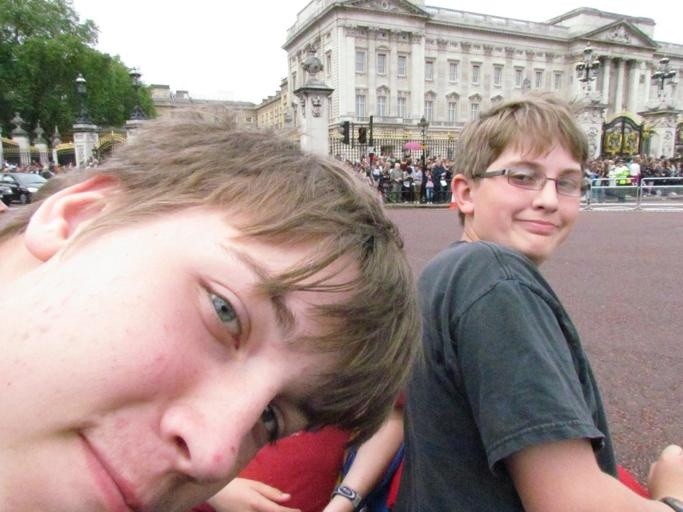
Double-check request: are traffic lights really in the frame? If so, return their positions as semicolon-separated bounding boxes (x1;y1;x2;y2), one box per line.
358;128;366;143
336;121;345;144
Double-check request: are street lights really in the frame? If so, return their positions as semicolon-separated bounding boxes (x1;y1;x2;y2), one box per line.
417;115;429;203
573;41;600;94
127;66;147;120
649;53;676;104
74;74;93;123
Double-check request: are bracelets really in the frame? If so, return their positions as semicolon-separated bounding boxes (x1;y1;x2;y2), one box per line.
330;483;365;509
661;498;682;511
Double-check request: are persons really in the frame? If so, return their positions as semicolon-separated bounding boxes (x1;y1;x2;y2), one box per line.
582;152;683;203
397;90;683;511
1;113;422;512
0;159;74;179
336;152;454;206
195;386;408;512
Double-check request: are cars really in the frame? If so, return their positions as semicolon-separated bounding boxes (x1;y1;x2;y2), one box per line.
0;173;47;203
0;186;16;206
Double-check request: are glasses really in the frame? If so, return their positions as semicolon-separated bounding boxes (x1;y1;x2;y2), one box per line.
477;167;592;197
